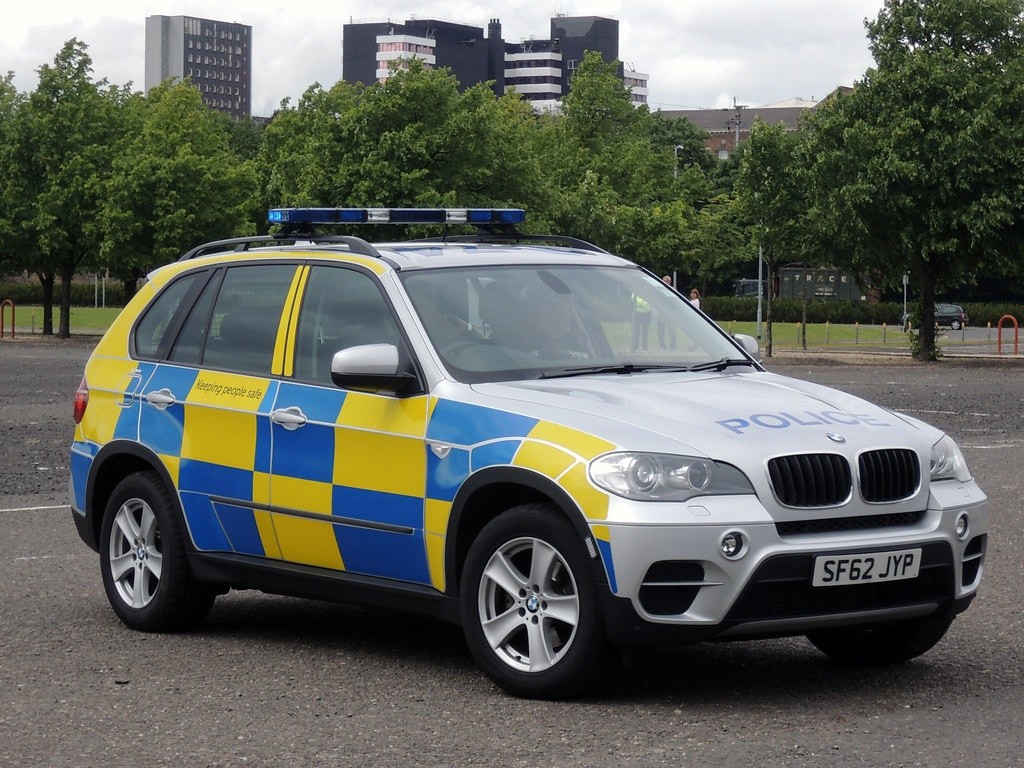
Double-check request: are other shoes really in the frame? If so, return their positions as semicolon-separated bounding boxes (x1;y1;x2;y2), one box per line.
630;349;649;353
671;347;677;352
657;348;666;351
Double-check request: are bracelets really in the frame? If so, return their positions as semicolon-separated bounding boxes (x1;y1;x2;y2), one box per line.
466;323;473;336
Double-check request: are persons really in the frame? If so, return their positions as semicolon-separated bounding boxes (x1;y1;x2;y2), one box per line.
501;288;589;363
390;283;488;348
629;275;700;351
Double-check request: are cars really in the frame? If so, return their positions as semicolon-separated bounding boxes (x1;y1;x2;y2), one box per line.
68;208;990;700
900;304;969;331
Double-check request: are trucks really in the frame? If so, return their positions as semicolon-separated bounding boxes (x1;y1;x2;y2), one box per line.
731;266;856;301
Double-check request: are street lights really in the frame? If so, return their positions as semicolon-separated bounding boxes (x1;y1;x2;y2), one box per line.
673;144;685;289
